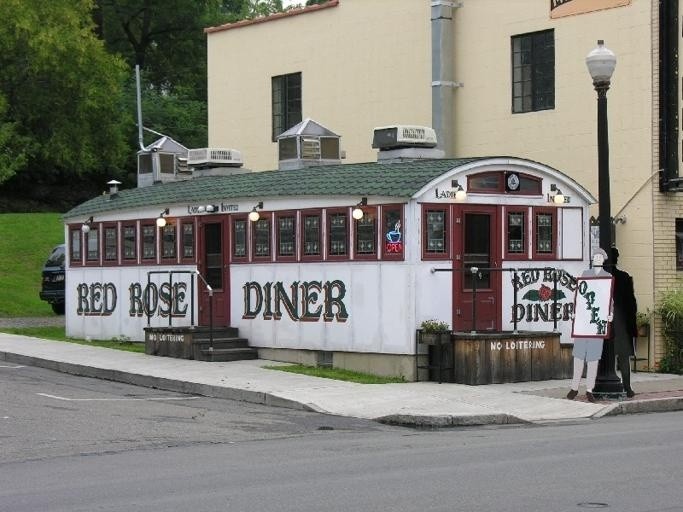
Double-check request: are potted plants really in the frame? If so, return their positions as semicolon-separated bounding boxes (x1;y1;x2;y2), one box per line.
420;317;452;346
635;311;649;336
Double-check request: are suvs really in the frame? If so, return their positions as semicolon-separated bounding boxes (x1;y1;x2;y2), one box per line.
40;240;99;315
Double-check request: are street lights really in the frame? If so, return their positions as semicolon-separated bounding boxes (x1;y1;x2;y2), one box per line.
583;35;625;402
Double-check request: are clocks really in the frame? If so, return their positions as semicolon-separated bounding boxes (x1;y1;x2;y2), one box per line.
504;170;520;192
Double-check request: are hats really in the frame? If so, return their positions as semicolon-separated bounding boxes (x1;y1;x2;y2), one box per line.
592;247;609;260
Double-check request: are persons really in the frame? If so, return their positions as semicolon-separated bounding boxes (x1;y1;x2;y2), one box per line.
601;248;638;400
567;245;613;401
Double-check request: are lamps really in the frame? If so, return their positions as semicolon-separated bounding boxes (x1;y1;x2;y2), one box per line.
80;216;92;234
197;205;218;212
450;179;467;202
351;197;367;220
550;183;564;204
155;208;168;227
248;201;263;222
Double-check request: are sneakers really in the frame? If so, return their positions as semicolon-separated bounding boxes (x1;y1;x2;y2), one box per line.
566;388;598;403
